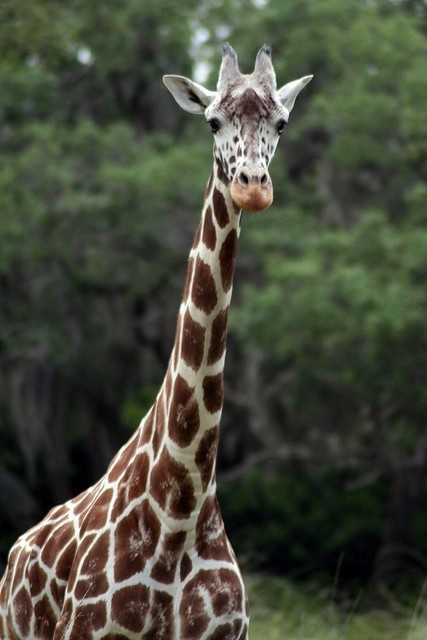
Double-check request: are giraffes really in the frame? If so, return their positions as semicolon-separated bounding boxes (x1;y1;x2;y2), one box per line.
0;41;318;639
